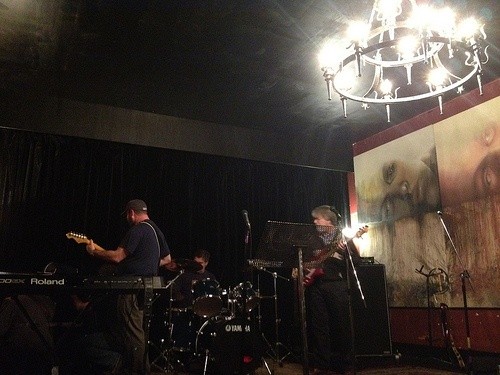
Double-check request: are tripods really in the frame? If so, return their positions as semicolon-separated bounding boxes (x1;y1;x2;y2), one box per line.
256;272;299;375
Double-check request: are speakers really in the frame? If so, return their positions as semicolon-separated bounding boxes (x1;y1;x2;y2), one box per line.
355;265;392;358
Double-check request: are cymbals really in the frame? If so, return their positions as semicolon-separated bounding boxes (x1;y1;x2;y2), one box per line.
174;259;204;272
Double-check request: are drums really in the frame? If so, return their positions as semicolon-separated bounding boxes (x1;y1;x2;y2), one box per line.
229;283;262;311
157;309;262;375
191;280;222;315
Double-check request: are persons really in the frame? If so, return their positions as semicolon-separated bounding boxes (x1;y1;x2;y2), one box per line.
86;199;172;375
180;249;215;297
303;205;360;375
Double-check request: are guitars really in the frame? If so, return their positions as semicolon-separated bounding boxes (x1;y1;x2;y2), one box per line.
293;226;369;285
66;233;106;252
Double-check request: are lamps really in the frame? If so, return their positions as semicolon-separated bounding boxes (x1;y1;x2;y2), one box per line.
317;0;490;122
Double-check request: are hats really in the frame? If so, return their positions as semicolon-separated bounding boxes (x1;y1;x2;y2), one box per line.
121;199;148;216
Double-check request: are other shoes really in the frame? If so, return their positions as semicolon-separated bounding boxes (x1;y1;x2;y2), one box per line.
312;368;329;375
344;368;352;375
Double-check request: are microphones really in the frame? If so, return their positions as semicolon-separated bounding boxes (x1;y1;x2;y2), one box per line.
247;259;266;272
241;209;251;230
